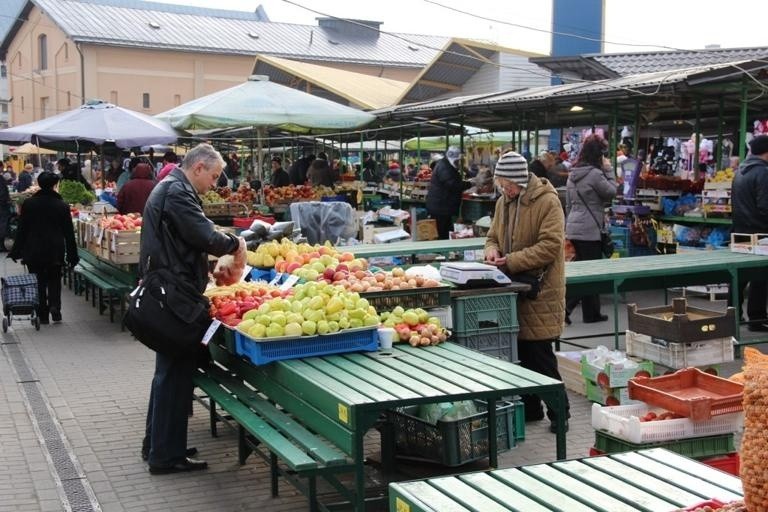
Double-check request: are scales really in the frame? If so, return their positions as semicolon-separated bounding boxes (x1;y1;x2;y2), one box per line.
439;261;513;289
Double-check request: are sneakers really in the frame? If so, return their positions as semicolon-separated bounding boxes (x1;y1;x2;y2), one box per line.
746;321;768;333
548;417;570;434
582;314;610;324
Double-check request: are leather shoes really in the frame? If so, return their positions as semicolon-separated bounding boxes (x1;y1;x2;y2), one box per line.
146;452;209;476
139;442;198;463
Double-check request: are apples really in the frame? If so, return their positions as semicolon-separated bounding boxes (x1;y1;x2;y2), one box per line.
215;185;317;203
606;396;620;406
317;253;369;286
636;370;651;378
97;212;142;233
208;288;292;327
598;372;609;388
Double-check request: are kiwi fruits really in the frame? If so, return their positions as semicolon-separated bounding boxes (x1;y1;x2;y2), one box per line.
399;414;489;458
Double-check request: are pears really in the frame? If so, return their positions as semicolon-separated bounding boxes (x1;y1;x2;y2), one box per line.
238;281;441;337
245;237;339;281
198;191;224;205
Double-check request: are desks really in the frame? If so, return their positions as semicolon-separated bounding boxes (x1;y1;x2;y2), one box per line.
553;251;767;359
333;237;487;258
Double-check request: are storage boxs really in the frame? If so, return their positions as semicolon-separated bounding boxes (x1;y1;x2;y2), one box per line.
581;352;719;406
77;220;140;264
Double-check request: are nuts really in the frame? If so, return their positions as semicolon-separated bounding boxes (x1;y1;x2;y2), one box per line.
674;358;768;512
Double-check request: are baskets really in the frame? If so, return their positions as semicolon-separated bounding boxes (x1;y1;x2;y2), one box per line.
386;293;527;469
589;367;745;459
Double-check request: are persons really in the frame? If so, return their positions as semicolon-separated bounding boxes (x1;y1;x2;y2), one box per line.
483;135;617;433
2;152;180;252
426;145;478;242
218;148;423;193
13;172;79;324
138;143;245;475
727;135;768;332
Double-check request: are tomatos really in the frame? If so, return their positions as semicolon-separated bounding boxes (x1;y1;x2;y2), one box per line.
640;412;684;422
70;207;79;216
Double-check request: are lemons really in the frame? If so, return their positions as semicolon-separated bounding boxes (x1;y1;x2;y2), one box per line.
705;168;735;183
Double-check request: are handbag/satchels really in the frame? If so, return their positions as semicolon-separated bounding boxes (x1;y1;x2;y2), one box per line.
598;229;615;259
120;263;213;356
504;272;544;301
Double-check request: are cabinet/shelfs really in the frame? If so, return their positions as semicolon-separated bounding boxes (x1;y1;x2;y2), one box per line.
59;246;139;333
192;330;565;512
389;445;746;512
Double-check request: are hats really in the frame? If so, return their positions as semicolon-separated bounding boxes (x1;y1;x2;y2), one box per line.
445;145;462;168
272;156;281;165
492;149;529;189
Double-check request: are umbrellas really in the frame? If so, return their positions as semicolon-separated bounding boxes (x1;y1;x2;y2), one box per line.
152;75;376;205
0;98;178;218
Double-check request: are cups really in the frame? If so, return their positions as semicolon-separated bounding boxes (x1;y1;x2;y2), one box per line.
379;326;396;351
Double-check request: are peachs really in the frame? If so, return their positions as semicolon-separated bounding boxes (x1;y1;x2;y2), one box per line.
397;323;446;346
335;267;441;311
